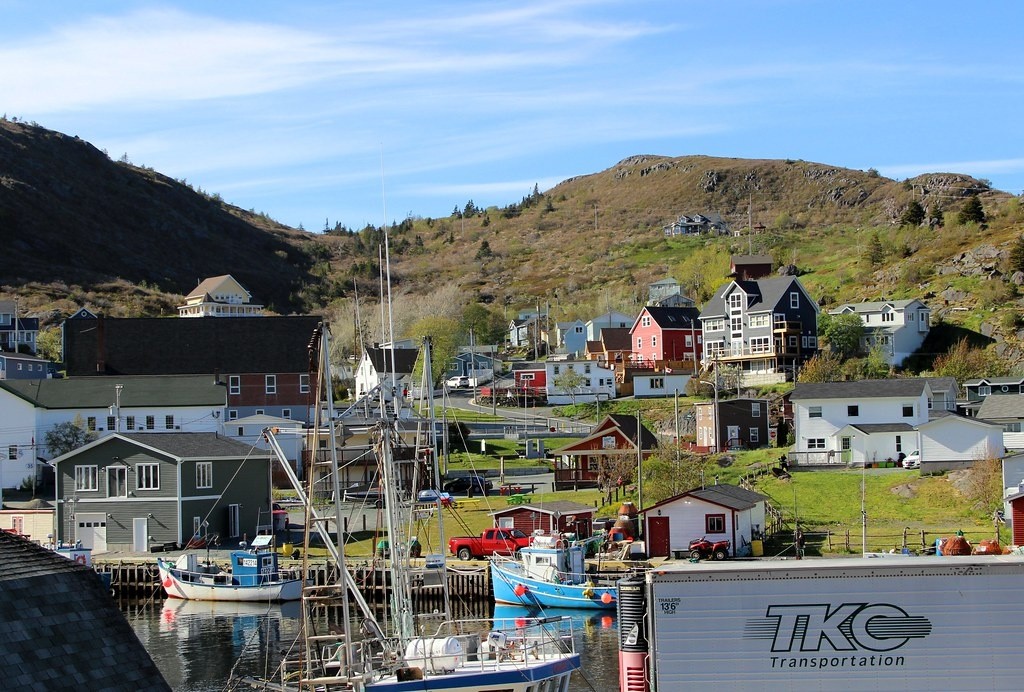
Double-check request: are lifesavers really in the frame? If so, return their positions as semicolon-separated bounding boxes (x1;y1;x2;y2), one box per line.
77;555;86;564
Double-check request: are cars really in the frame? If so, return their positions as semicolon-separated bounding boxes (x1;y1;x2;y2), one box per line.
901;449;921;468
444;477;493;494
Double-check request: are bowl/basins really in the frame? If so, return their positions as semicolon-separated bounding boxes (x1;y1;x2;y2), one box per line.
976;546;987;552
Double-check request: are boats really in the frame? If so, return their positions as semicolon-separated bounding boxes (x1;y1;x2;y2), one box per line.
157;430;314;603
491;487;622;610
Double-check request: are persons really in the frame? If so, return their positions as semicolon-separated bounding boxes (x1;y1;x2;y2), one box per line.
780;454;789;473
617;475;623;490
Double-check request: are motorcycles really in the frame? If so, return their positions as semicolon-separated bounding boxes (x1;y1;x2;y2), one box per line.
689;536;730;561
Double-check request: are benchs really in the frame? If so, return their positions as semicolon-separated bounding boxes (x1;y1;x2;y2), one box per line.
498;486;522;496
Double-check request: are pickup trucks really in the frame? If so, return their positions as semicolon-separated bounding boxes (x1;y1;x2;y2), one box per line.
449;528;532;559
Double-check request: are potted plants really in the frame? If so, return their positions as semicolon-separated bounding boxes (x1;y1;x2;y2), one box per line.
865;449;872;468
885;456;894;468
872;450;878;468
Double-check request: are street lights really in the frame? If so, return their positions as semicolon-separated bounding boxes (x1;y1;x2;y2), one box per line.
699;381;721;453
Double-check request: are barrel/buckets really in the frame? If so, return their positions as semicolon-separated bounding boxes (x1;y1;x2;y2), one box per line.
609;527;627;541
935;537;947;556
613;533;624;541
283;542;293;557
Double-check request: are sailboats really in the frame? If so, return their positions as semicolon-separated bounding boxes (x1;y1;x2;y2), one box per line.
224;188;580;692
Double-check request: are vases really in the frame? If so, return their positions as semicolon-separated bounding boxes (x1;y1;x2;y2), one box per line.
878;461;886;468
